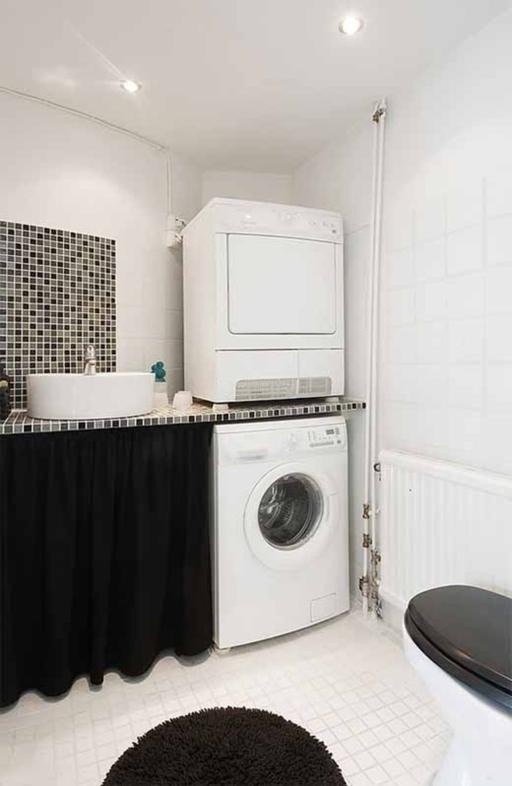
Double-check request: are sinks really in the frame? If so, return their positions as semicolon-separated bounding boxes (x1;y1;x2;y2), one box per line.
25;372;156;421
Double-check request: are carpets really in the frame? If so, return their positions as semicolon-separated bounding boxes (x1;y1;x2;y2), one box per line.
100;704;348;785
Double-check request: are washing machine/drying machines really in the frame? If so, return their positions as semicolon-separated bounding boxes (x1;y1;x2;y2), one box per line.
179;197;346;412
207;415;353;655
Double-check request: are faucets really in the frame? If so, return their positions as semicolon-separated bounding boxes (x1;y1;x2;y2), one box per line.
83;345;97;376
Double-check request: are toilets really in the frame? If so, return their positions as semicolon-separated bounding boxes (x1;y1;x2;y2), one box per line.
398;585;511;786
